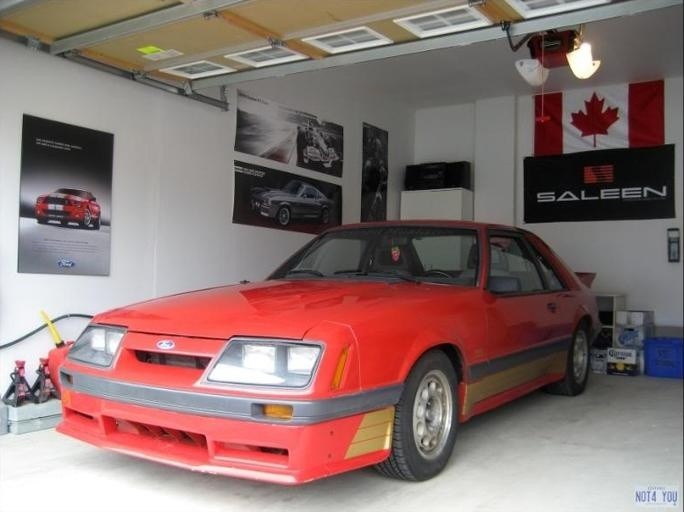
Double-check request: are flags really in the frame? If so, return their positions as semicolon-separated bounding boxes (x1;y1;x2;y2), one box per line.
533;79;665;155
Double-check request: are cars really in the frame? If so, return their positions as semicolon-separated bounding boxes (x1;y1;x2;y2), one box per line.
297;120;340;169
52;217;601;489
249;182;338;228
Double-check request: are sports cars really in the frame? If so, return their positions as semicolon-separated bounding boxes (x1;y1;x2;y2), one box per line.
36;187;103;230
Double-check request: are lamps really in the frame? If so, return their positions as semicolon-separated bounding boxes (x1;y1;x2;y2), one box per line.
503;25;601;85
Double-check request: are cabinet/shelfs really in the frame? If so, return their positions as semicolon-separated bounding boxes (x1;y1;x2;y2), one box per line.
594;292;626;352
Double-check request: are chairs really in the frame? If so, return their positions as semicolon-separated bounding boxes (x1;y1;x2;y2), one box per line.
372;236;419;276
459;243;521;293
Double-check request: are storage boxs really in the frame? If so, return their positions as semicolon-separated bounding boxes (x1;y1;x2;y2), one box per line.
642;338;684;379
605;348;644;376
616;309;653;328
615;324;654;348
7;398;62;435
589;348;607;374
